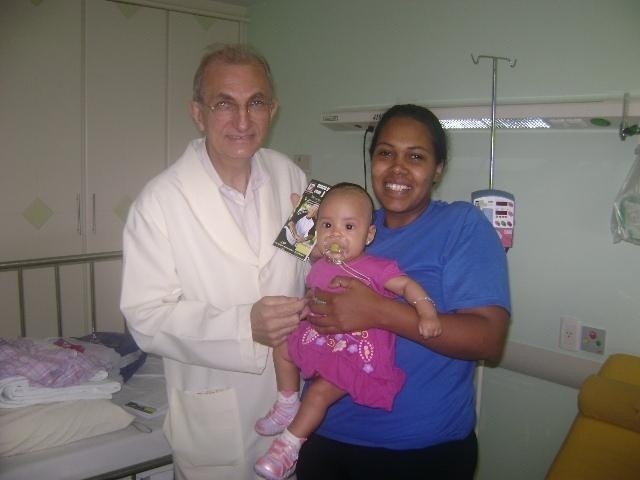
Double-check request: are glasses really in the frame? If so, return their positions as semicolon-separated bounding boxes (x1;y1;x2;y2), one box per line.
201;98;273;113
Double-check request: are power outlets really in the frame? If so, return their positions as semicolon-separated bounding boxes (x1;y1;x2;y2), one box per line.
560;320;579;352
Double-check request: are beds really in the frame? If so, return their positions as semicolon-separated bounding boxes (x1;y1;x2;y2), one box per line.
543;353;639;479
0;252;174;480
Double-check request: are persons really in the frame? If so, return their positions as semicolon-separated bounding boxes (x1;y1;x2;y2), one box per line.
291;103;510;478
254;183;442;480
279;204;321;250
115;46;310;478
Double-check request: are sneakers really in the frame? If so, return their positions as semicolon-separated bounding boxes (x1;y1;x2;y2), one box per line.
255;399;301;435
254;440;299;480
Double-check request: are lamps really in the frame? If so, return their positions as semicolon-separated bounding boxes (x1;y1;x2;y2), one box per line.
317;99;640;134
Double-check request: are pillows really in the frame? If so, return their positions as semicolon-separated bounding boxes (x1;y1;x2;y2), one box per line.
1;399;135;457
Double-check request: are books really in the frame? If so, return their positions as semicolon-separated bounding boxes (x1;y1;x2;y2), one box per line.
270;178;333;264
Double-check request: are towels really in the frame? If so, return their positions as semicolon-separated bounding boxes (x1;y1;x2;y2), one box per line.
0;338;124;410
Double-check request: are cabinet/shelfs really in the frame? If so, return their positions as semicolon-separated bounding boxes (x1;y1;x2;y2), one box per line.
1;1;166;337
166;1;248;168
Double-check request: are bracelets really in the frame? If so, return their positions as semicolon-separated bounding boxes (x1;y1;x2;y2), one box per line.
411;297;437;308
